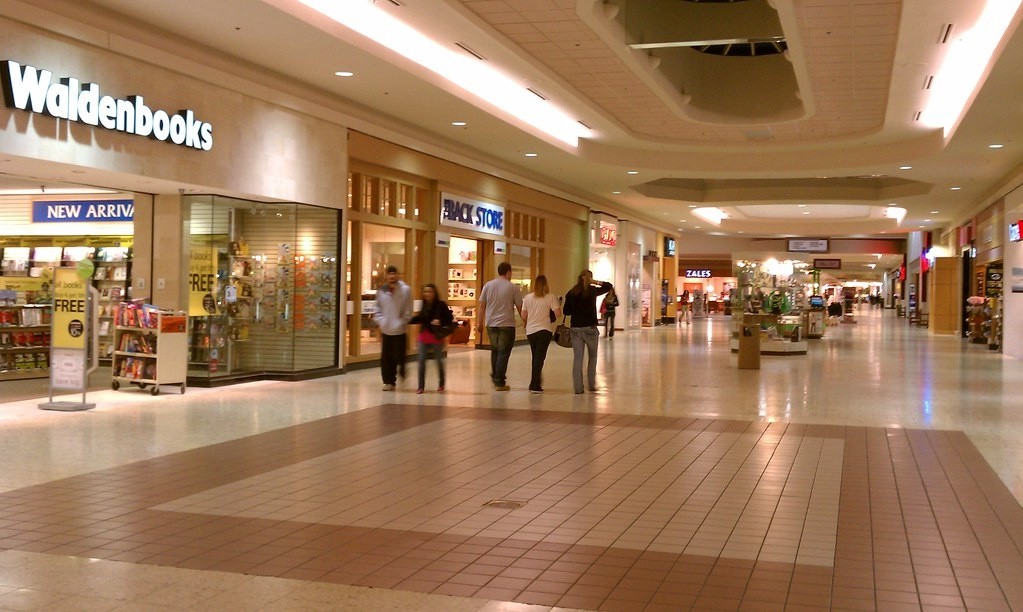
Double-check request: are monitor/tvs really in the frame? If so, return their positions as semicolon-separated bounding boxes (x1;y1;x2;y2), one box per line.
810;296;823;307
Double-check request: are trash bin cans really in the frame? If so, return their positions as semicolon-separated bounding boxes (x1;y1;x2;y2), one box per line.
738;323;761;369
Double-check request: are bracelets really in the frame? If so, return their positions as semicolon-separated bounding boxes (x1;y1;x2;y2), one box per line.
596;280;599;285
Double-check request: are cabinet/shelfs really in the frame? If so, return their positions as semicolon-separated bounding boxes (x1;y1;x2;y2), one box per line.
112;301;188;394
93;247;134;361
0;294;53;380
189;316;240;378
446;262;477;319
226;253;265;325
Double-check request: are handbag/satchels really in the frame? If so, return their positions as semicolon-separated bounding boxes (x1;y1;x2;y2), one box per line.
600;299;607;314
554;314;574;348
426;302;459;339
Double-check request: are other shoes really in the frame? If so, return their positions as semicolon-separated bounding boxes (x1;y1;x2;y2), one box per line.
531;389;544;394
438;386;444;391
496;386;510;391
382;384;395;391
416;387;425;393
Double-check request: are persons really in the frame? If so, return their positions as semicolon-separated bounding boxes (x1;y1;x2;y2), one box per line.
520;275;561;394
679;290;691;324
563;270;612;394
604;288;619;339
407;283;453;394
810;314;816;332
477;262;523;391
869;294;880;306
373;266;412;391
756;287;763;297
